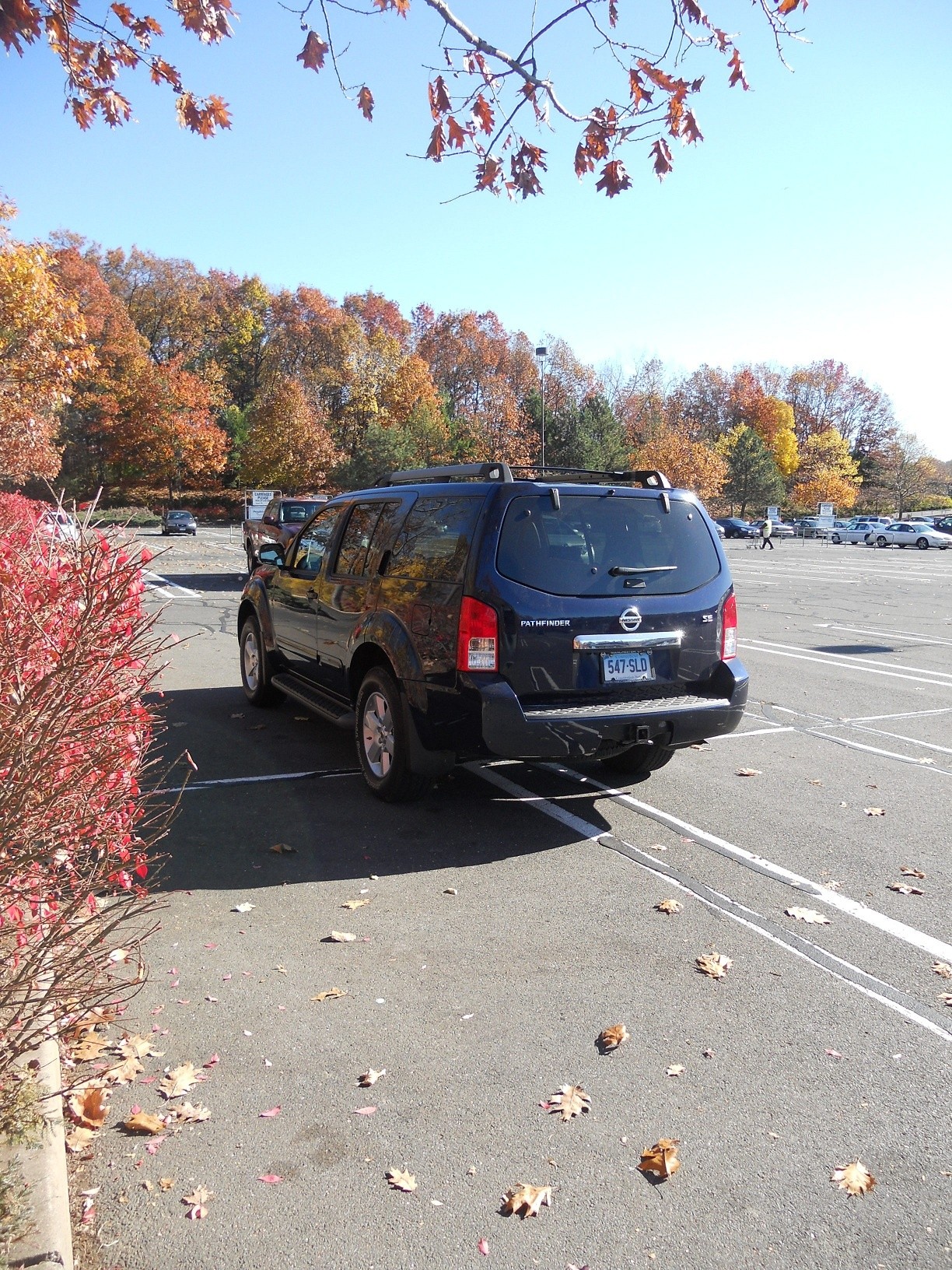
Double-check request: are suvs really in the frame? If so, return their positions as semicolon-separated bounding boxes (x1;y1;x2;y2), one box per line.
235;462;750;807
715;517;761;539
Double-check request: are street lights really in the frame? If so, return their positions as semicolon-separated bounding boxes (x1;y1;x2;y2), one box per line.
535;347;549;478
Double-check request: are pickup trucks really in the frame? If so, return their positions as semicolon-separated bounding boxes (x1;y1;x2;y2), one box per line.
242;497;329;576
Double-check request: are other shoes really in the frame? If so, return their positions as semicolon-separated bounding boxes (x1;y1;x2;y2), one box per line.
769;548;773;550
761;548;764;549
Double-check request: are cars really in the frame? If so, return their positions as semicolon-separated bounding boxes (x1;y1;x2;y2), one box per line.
827;521;887;545
710;517;725;541
44;511;80;551
161;510;198;537
786;514;952;538
749;519;795;540
869;521;952;550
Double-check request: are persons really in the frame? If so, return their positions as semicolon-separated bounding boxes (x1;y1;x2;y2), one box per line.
759;516;774;550
